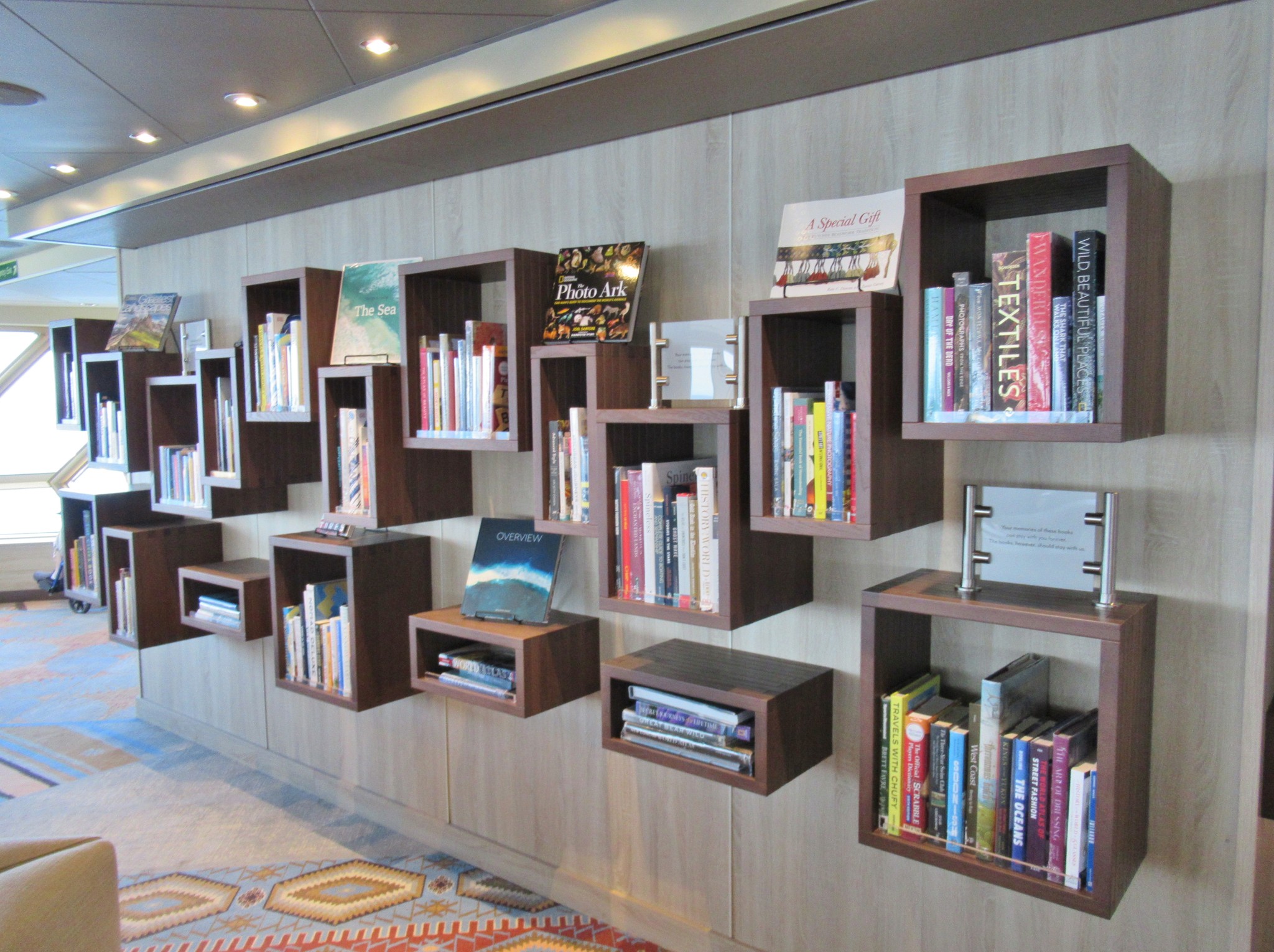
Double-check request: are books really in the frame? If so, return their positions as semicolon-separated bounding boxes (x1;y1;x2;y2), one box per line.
194;591;241;630
440;644;516;700
542;242;649;345
158;442;204;508
336;407;372;517
329;258;424;365
611;456;720;614
769;379;856;522
210;377;237;472
460;518;565;623
69;509;97;598
547;407;590;523
254;313;305;413
923;230;1106;425
416;319;509;432
876;653;1099;896
621;686;754;776
105;293;181;353
283;579;351;698
115;568;134;637
60;351;79;420
770;188;906;298
91;393;126;459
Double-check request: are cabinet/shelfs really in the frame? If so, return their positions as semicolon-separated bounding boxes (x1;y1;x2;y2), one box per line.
269;526;431;712
530;342;813;630
81;352;182;473
59;483;185;607
749;292;945;542
398;247;558;453
145;347;323;519
318;364;473;530
49;318;116;431
178;557;273;643
901;146;1171;439
858;568;1157;919
409;603;600;719
240;267;342;422
102;519;224;649
600;639;836;795
